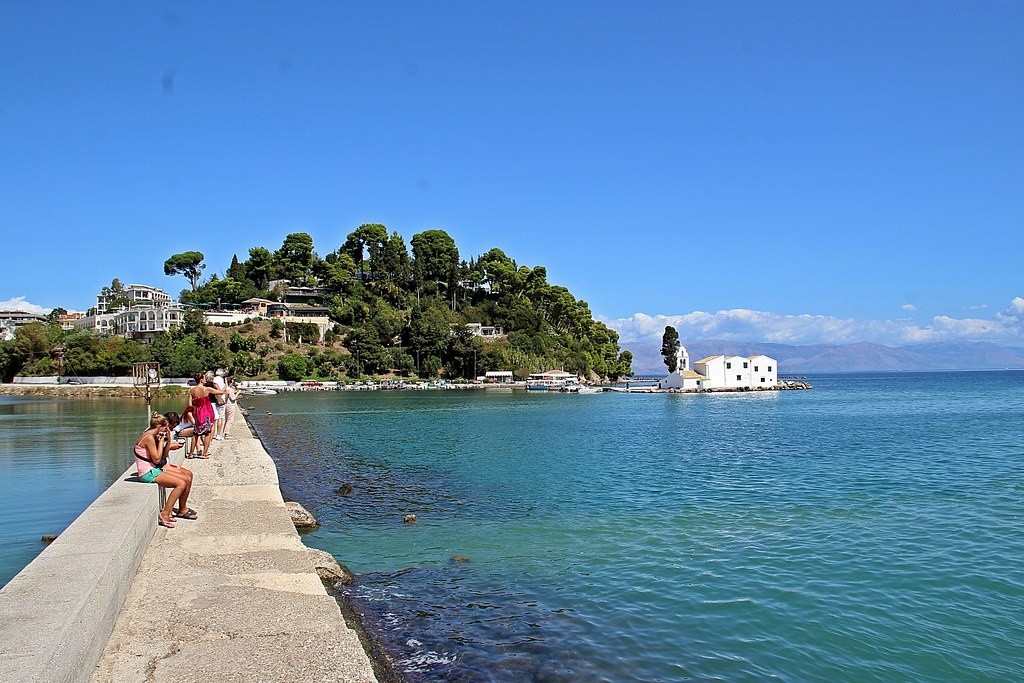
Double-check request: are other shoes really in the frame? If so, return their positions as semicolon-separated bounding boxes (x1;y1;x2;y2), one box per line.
197;450;202;456
201;456;209;459
224;434;232;439
188;454;193;459
213;434;222;440
159;509;178;528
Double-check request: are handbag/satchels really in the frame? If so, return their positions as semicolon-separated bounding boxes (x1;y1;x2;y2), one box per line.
193;418;212;435
154;456;167;468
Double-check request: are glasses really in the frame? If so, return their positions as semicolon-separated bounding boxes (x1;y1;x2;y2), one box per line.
158;432;168;441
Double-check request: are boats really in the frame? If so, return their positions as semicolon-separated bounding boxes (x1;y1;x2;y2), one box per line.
238;379;603;395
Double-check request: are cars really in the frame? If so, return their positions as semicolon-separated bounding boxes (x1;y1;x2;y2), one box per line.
187;380;197;386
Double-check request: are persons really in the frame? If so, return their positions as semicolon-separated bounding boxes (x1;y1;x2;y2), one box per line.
205;370;226;439
223;377;239;439
213;368;230;440
188;372;226;459
143;410;198;520
134;411;189;528
175;404;211;455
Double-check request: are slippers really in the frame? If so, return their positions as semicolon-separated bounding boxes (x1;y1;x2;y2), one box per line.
176;508;197;520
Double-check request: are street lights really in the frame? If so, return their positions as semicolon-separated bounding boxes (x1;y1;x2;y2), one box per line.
133;361;160;428
